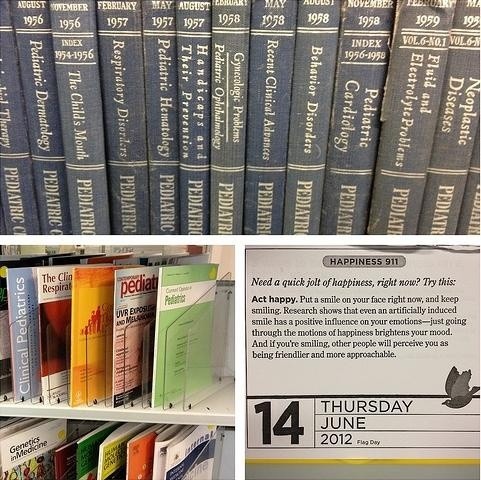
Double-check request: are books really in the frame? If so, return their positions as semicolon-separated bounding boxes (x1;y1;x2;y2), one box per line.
0;252;218;409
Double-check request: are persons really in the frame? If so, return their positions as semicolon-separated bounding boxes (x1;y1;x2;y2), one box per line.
0;416;218;480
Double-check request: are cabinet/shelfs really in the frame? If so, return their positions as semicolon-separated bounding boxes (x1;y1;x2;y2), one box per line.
0;236;481;480
